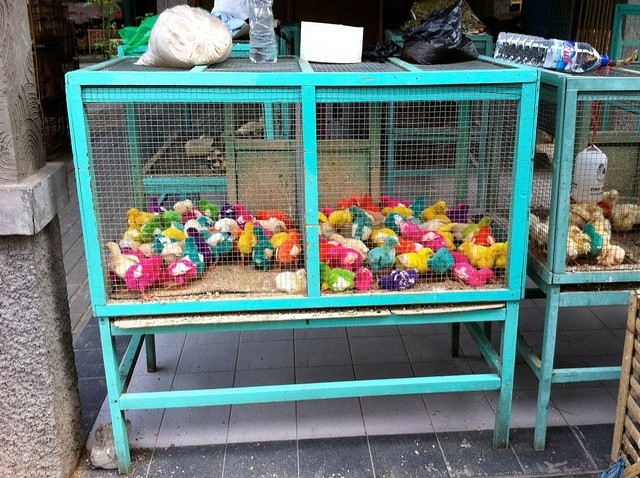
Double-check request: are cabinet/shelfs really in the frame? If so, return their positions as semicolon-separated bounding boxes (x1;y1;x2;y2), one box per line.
65;54;542;476
601;2;639;132
482;55;640;452
384;28;493;236
117;26;299;195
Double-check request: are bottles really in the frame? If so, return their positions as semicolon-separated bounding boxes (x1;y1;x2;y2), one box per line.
248;0;279;63
493;31;610;73
